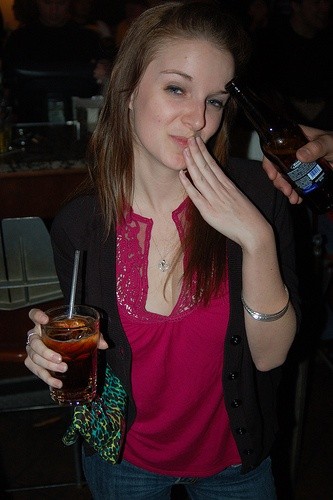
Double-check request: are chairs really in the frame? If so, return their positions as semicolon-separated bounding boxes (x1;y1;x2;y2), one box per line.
0;216;87;493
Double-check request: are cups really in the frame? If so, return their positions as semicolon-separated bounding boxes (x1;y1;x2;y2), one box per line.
40;304;100;406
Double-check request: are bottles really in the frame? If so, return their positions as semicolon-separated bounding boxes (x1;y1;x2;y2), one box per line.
224;77;333;214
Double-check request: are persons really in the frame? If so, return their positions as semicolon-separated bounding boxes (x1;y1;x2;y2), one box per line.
23;0;298;500
0;0;43;123
261;125;333;207
278;0;333;121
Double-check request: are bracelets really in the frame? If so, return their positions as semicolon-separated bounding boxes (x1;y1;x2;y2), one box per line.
240;282;290;322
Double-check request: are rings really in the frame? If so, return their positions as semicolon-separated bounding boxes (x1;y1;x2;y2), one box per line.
26;333;41;346
